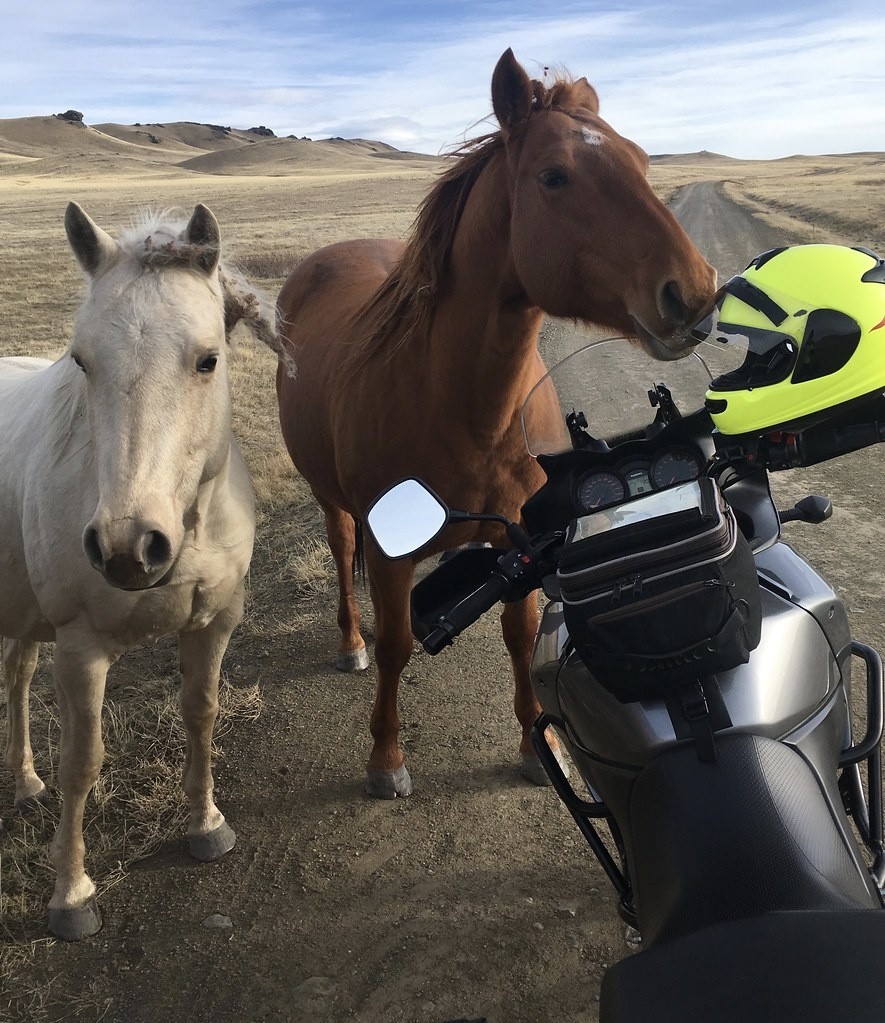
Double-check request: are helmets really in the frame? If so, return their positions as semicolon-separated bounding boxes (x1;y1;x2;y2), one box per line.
692;244;885;470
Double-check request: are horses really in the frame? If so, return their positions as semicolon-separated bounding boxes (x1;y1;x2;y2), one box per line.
0;201;256;941
274;47;718;800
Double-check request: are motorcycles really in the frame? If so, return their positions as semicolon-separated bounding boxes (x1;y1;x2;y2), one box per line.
362;294;882;1023
